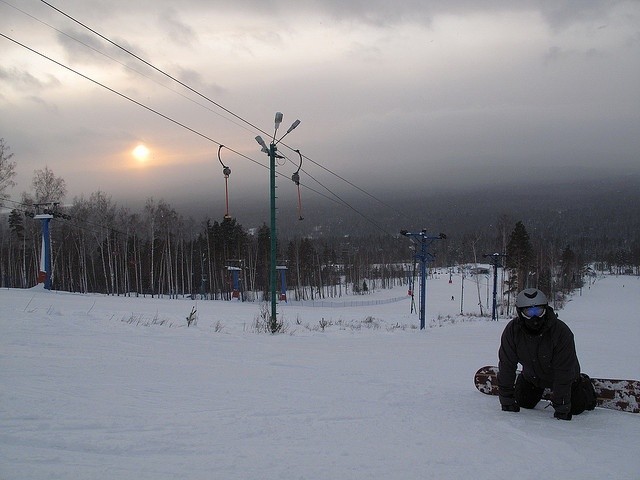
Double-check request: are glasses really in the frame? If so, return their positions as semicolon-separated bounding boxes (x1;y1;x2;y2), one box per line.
519;304;547;320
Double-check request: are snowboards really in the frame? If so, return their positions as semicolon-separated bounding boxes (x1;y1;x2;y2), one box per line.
474;366;640;414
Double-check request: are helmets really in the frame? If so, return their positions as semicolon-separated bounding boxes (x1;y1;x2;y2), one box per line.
514;287;547;332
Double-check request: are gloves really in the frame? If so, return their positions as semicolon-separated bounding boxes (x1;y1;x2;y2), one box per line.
553;402;573;421
499;386;520;412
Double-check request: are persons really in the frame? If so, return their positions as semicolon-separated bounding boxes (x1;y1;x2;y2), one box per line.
497;287;596;421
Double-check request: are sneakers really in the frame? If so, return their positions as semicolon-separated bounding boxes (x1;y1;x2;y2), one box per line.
579;373;597;411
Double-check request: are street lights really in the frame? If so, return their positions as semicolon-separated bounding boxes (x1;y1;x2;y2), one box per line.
254;111;301;334
